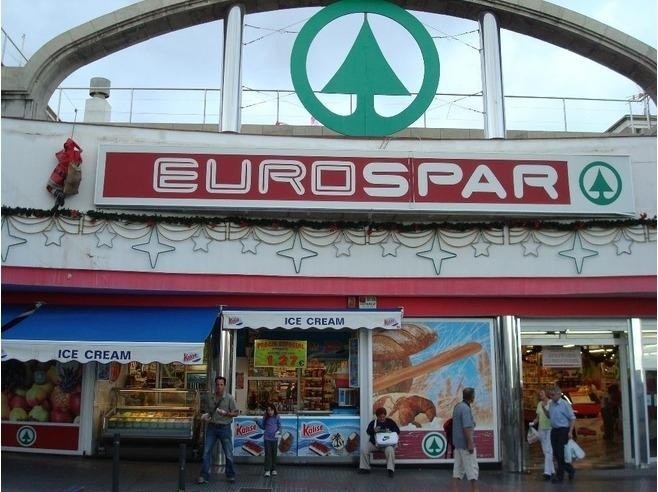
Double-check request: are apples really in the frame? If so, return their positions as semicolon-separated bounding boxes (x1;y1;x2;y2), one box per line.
51;408;74;423
70;392;81;415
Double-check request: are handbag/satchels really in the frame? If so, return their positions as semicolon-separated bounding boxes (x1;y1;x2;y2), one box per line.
443;418;452;444
375;432;399;447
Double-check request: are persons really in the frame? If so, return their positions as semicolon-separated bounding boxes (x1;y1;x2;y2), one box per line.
355;406;401;480
196;375;239;485
252;403;282;479
442;385;481;492
525;382;587;486
45;137;85;212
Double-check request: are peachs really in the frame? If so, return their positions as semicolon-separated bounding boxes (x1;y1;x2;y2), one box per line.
1;383;53;421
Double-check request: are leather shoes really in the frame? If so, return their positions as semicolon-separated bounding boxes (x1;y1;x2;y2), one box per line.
542;470;575;483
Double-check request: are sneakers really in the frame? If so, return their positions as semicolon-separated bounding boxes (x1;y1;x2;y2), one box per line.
356;469;370;474
272;471;277;475
264;471;270;476
387;470;394;479
227;478;235;482
198;477;207;483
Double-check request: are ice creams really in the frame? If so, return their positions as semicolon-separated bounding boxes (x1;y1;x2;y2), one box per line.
332;433;345;455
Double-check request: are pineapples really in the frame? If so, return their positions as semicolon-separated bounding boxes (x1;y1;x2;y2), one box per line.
51;367;82;412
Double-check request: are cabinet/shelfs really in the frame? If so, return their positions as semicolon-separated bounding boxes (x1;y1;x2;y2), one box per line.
249;366;331;415
99;404;194;438
